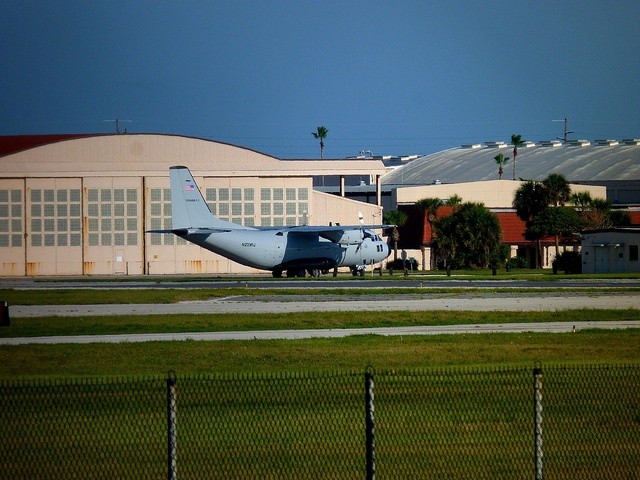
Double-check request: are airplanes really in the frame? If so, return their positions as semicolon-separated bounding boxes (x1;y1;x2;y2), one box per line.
143;165;399;280
